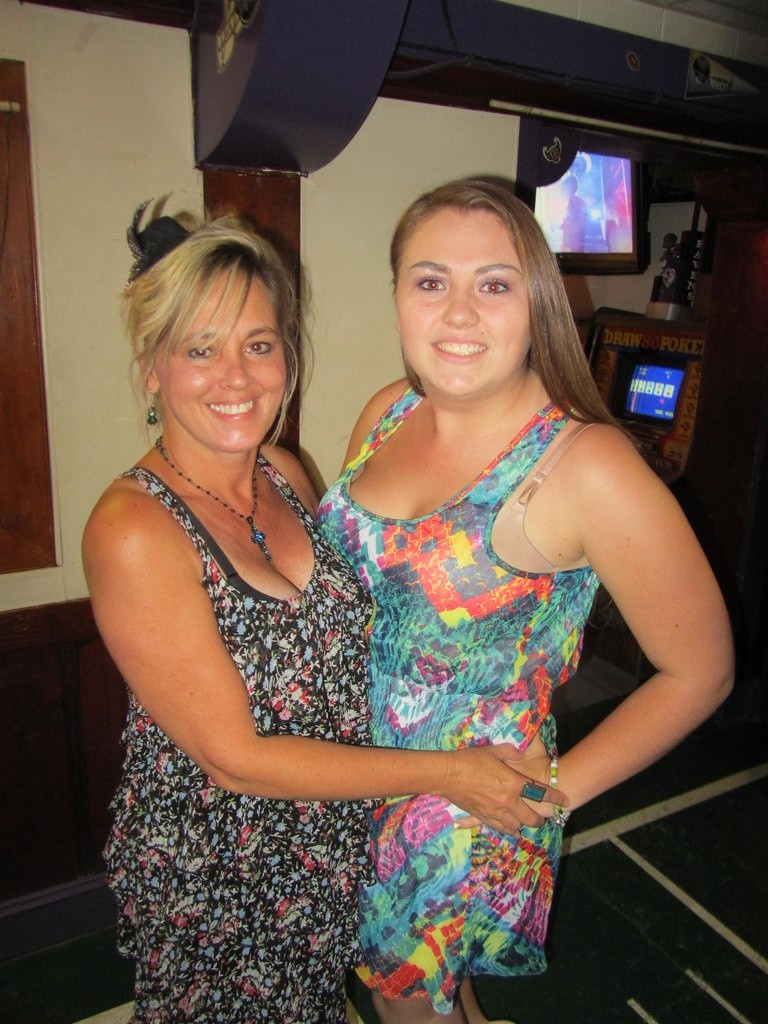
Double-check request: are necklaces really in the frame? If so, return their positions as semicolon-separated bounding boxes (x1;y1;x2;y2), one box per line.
157;438;272;563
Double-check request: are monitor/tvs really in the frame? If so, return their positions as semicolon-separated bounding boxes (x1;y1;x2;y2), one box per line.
623;363;684;430
534;141;654;275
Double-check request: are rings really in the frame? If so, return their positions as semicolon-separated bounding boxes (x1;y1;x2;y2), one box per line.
520;782;545;803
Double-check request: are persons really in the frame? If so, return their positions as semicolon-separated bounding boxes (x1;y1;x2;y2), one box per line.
660;233;696;280
316;178;734;1024
81;192;568;1024
559;174;586;252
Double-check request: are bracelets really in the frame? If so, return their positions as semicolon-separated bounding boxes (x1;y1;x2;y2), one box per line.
550;756;573;833
